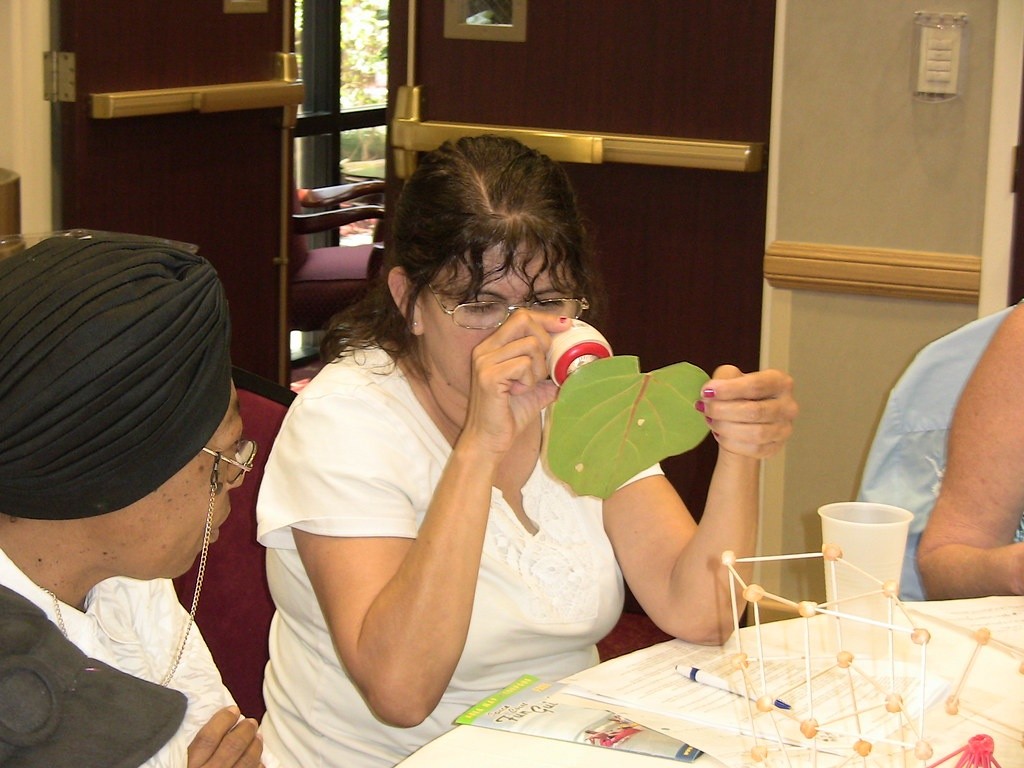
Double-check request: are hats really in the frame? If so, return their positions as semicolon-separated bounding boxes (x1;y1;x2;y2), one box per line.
1;229;233;519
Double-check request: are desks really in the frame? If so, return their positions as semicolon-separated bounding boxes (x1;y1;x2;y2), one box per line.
389;594;1024;767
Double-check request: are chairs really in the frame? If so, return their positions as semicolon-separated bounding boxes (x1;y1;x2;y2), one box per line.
172;363;299;722
288;180;387;332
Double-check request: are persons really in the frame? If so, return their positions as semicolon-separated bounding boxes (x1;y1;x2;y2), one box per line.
914;299;1024;599
257;134;798;767
0;229;265;767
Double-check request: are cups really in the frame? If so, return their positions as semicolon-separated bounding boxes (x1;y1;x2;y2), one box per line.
817;501;914;654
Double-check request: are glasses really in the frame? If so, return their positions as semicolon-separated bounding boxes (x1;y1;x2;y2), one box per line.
199;437;258;487
426;284;590;331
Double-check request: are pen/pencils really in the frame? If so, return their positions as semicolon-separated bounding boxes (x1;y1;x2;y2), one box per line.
673;664;792;710
